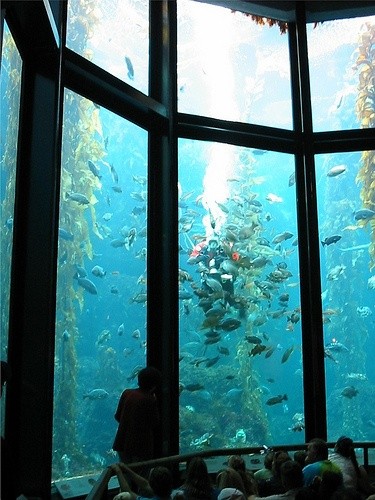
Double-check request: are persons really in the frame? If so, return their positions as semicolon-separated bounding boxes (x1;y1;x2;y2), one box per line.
112;367;160;477
106;435;375;500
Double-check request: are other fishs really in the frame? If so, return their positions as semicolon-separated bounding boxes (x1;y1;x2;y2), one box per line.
0;53;375;455
